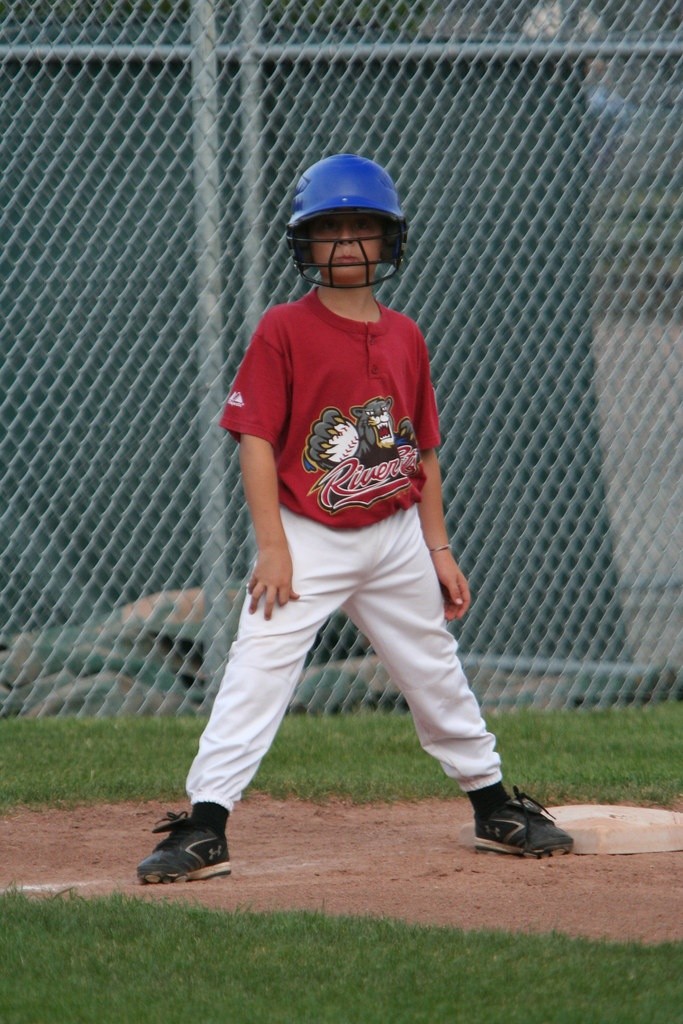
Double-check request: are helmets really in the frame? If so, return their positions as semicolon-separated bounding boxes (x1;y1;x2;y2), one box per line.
287;154;406;260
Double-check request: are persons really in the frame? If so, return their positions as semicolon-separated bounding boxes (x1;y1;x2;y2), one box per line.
135;153;575;885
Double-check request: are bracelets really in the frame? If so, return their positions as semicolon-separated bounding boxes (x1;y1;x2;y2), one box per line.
430;544;451;553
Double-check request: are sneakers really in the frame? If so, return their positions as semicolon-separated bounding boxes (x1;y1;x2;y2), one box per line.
474;786;573;859
137;812;232;884
